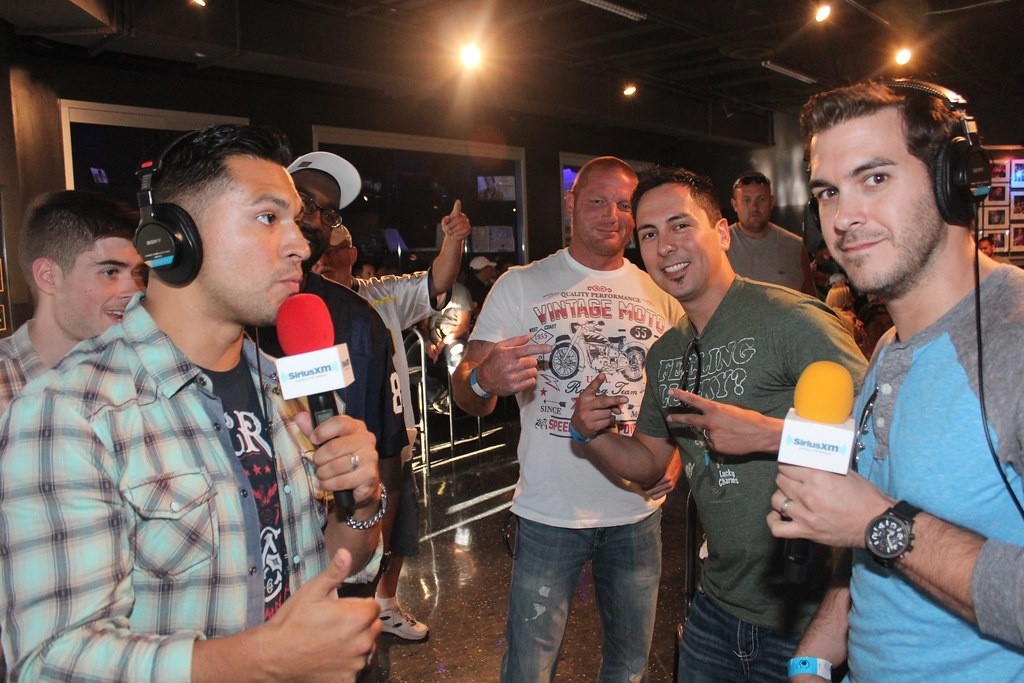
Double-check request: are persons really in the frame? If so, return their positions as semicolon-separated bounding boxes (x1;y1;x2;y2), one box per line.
0;190;147;419
764;79;1024;683
811;239;894;360
0;123;386;683
312;196;471;642
450;156;691;683
572;167;870;683
352;252;519;416
725;171;818;299
979;237;1012;265
239;150;410;598
989;187;1005;201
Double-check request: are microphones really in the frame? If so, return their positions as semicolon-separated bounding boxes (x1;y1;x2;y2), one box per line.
275;293;356;509
778;362;856;564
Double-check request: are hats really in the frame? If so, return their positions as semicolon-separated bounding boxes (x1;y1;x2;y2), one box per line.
285;151;362;210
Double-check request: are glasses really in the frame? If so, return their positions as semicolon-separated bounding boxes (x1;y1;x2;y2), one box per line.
852;387;878;474
676;337;702;407
502;513;520;561
298;192;344;229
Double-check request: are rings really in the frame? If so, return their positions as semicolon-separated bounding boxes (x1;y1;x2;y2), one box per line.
781;498;792;517
349;454;359;471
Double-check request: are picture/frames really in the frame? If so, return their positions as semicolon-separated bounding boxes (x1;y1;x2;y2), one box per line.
972;158;1024;262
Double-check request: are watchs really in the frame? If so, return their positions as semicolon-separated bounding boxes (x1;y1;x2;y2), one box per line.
864;500;923;569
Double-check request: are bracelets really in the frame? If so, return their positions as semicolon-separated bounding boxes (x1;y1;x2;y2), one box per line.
344;482;389;530
470;367;492;399
569;423;590;443
787;657;832;681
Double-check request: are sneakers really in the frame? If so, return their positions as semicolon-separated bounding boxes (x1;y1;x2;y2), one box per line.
374;603;429;642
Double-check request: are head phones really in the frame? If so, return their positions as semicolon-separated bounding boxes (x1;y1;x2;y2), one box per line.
131;129;203;289
808;78;993;233
